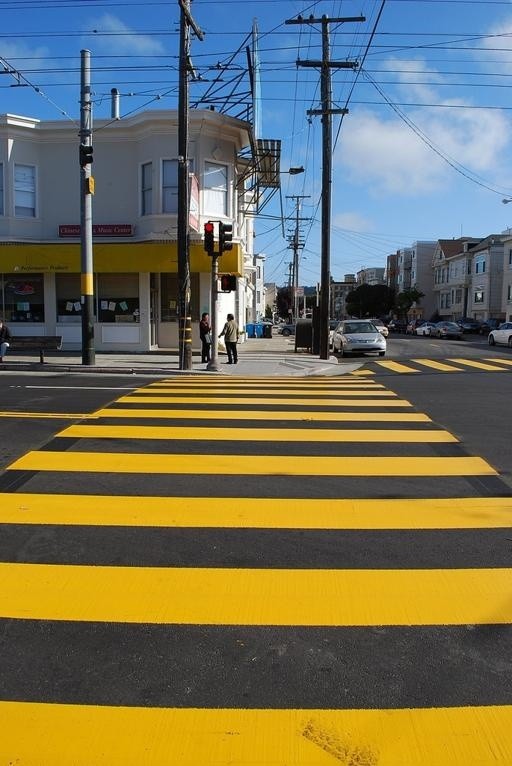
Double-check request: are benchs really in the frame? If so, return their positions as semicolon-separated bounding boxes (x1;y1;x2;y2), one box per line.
6;336;62;364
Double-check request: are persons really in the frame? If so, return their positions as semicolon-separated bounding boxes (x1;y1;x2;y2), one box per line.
199;312;211;363
218;313;239;364
0;318;15;363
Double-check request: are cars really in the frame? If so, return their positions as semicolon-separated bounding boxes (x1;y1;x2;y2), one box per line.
276;324;296;336
329;317;512;358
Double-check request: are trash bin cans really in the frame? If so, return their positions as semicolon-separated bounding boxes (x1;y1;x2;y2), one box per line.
246;322;273;338
294;318;313;353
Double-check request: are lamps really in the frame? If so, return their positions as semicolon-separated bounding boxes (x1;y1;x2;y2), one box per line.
13;242;20;272
237;165;305;176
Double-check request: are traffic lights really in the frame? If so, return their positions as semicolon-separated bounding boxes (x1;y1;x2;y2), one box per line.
204;223;214;252
78;143;94;167
218;222;233;252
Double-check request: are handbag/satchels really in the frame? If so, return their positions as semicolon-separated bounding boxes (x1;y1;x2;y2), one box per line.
204;333;212;344
